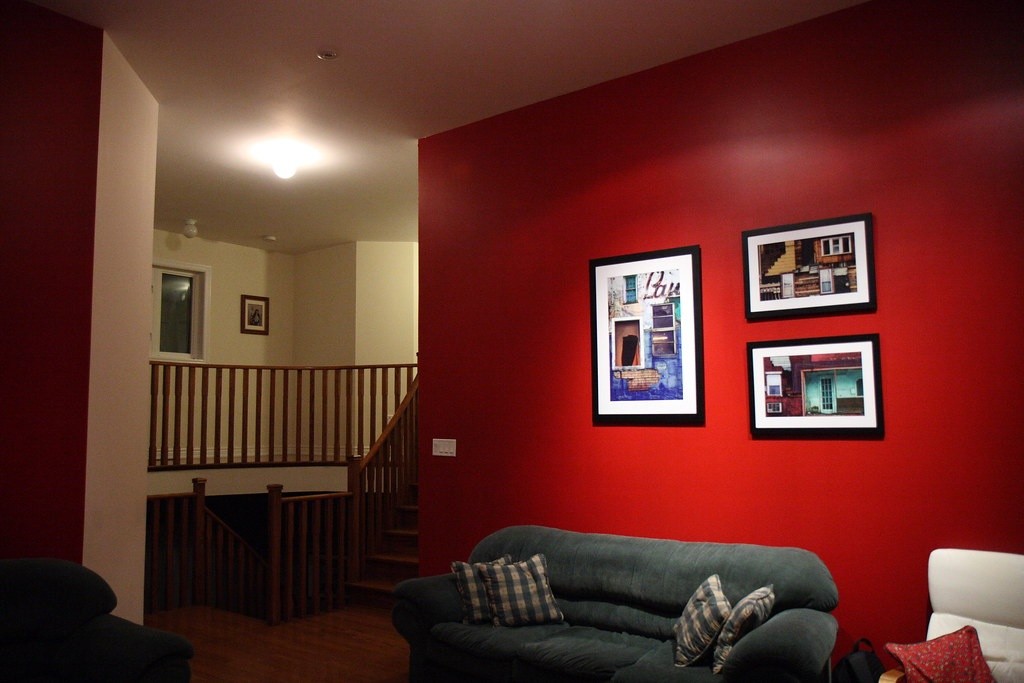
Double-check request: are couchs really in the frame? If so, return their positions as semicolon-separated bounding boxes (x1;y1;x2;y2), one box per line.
0;558;195;683
391;526;839;683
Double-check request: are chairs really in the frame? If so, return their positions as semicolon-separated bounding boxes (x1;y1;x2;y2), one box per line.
880;548;1024;683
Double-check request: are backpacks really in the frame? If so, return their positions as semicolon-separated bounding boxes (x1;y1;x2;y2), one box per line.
832;638;885;683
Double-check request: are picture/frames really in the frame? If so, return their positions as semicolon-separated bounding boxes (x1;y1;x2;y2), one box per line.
588;244;705;428
241;295;269;335
745;333;885;440
741;212;877;323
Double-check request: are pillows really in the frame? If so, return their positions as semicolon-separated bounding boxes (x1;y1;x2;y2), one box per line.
672;573;732;667
885;625;998;683
449;554;514;625
473;553;564;627
713;584;775;677
